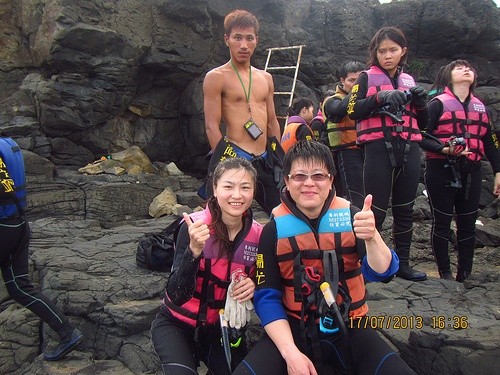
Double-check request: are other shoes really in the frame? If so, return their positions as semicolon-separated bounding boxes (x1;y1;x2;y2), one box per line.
44;327;84;361
439;269;473;283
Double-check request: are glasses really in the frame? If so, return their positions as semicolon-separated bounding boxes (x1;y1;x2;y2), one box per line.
288;172;331;181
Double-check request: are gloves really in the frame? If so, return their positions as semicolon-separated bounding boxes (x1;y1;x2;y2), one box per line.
274;165;286;190
377;90;407;113
409;85;428;108
223;269;255;330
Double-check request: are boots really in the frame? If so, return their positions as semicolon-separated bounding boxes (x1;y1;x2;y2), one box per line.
394;248;427;281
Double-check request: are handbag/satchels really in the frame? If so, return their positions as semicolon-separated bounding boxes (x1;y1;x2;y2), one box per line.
135;216;182;273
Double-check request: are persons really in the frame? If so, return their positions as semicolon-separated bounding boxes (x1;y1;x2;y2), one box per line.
203;9;285;219
0;135;84;360
419;58;500;289
151;157;265;375
282;99;315;153
234;140;416;375
348;25;428;282
309;90;335;145
321;60;371;210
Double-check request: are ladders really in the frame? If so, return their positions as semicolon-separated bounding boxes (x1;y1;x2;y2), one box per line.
264;45;306;141
